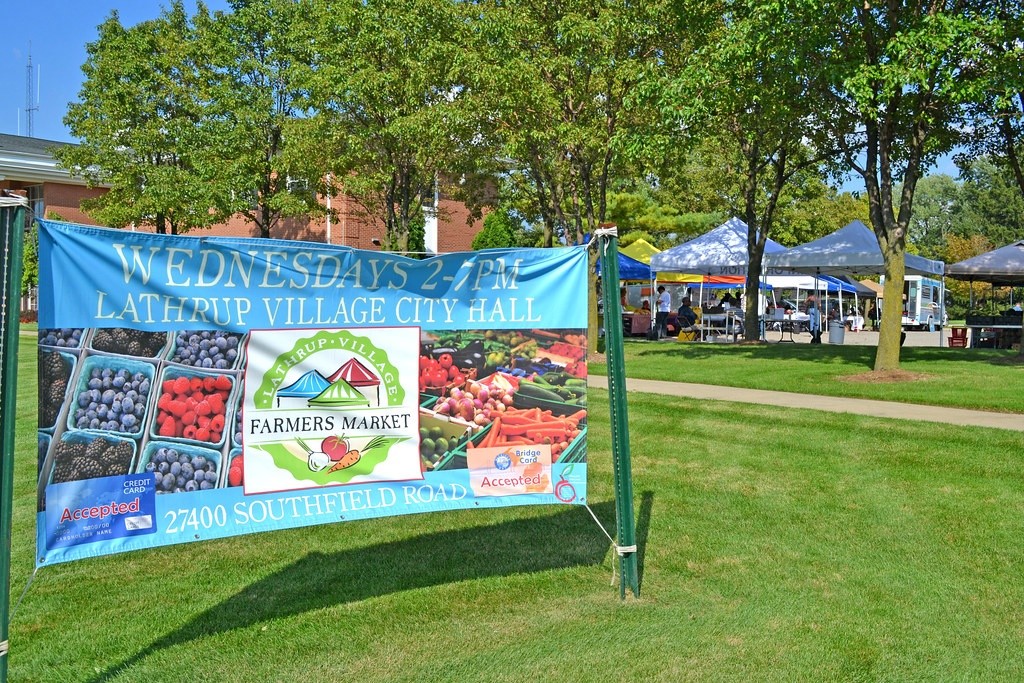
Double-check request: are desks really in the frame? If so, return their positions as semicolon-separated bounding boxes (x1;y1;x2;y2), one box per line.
622;314;651;337
764;314;810;344
964;324;1022;349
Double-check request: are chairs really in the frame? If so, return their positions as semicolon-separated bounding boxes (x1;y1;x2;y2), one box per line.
675;315;701;341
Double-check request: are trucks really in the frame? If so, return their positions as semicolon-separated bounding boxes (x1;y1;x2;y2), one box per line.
876;274;950;332
625;282;720;312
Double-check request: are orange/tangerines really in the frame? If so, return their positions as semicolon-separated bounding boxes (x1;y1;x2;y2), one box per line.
484;330;537;366
418;425;460;468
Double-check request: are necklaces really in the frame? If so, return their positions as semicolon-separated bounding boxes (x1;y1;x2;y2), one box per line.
678;297;708;342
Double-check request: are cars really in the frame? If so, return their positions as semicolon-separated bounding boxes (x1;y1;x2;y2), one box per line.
767;299;851;332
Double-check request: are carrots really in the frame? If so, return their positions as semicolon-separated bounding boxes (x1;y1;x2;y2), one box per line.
531;329;589;347
467;406;587;462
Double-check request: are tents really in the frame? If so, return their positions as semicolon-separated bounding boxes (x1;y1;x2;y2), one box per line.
944;241;1024;350
576;215;945;346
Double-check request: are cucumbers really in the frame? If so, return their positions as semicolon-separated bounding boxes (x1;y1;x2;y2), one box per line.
518;373;587;406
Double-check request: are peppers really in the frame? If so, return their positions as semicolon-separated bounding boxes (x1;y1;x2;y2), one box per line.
433;332;511;354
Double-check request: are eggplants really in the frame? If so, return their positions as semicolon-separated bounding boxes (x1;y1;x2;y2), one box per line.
420;339;488;374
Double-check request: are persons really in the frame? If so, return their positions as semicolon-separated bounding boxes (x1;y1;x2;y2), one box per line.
784;305;796;330
715;295;719;302
718;293;732;308
766;303;773;329
641;300;651;311
831;304;838;322
686;288;699;306
868;300;881;330
843;310;851;326
656;286;670;338
1014;302;1022;311
849;307;860;315
724;297;745;335
980;299;991;341
804;290;820;331
620;287;626;307
735;292;741;305
774;302;783;330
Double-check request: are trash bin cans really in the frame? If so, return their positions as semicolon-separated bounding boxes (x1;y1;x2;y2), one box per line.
829;320;844;346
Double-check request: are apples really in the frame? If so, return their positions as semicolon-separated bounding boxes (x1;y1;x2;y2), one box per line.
418;353;466;391
